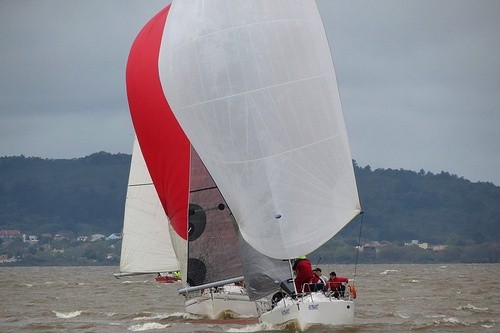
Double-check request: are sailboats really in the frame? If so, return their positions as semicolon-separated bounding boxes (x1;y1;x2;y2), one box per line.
157;0;363;333
126;3;296;321
114;133;182;283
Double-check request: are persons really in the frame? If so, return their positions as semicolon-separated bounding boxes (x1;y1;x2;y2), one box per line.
157;270;181;279
292;255;312;293
312;268;329;290
325;271;349;299
309;271;326;292
